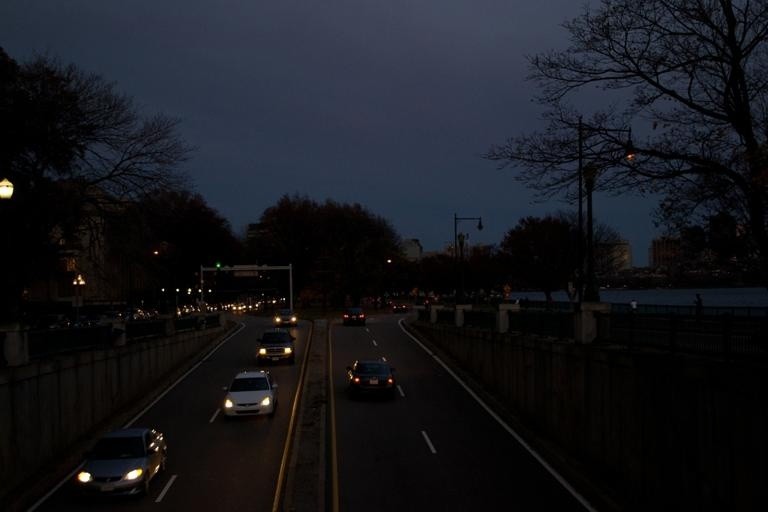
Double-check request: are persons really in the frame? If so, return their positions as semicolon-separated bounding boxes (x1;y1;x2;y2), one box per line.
630;299;638;314
691;292;704;316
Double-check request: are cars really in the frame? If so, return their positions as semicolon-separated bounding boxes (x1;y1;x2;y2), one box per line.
391;300;414;313
46;294;289;331
342;306;368;327
73;425;169;500
273;307;299;328
218;368;280;420
343;357;396;396
256;326;298;366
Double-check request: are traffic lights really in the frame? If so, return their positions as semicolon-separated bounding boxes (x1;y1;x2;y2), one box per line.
216;262;221;271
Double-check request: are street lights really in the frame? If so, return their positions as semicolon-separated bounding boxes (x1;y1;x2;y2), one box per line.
72;271;88;322
578;121;640;437
158;287;214;308
0;175;18;205
454;216;485;260
